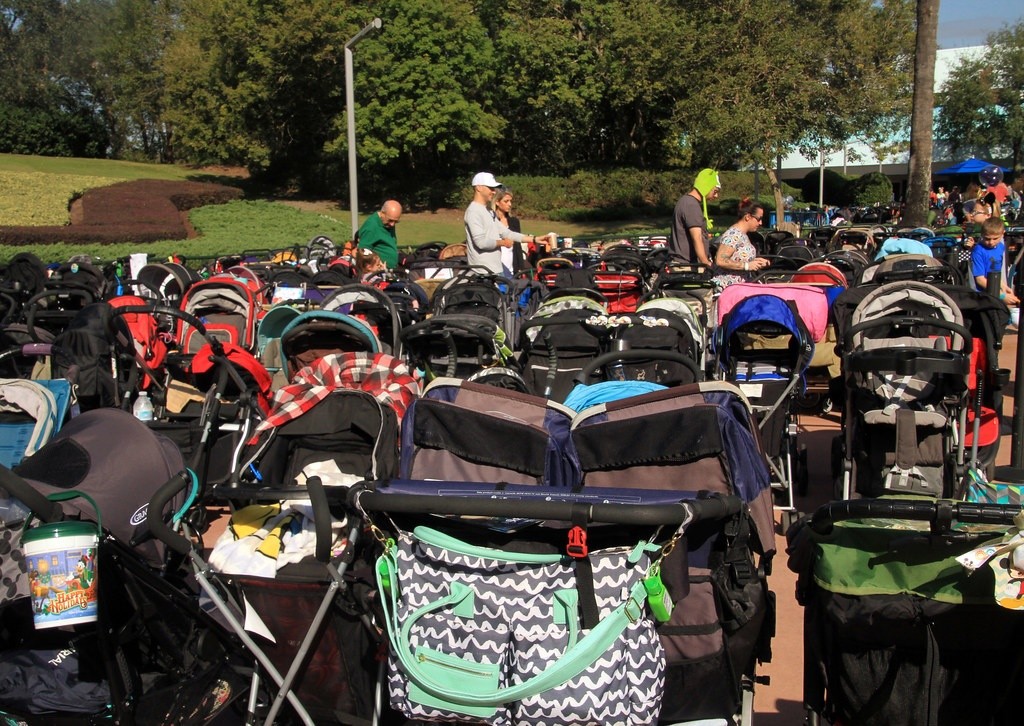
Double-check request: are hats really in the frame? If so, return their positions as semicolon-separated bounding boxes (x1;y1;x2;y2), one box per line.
471;172;502;188
694;168;721;230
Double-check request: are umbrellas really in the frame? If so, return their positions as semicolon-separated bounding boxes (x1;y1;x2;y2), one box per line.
934;157;1012;181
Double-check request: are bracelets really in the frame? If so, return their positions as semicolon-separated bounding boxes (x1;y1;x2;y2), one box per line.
1000;292;1006;301
533;236;536;244
744;262;748;271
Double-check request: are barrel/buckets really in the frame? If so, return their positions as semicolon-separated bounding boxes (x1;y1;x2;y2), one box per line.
564;238;573;248
20;490;103;630
1010;308;1020;329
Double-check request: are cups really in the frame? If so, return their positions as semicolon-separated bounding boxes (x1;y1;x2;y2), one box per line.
548;232;558;249
994;368;1011;384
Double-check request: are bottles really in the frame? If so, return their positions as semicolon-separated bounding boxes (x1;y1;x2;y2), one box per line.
378;562;400;599
643;576;673;621
133;391;153;421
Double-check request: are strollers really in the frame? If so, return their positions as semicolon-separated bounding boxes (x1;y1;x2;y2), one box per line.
0;200;1024;726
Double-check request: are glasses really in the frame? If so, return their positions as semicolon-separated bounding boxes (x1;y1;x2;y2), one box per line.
752;215;761;222
971;210;990;216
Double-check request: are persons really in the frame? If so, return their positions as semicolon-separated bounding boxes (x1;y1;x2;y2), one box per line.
355;200;402;269
355;248;386;282
489;185;525;278
464;172;551;275
669;168;721;266
929;181;1024;225
963;197;1020;331
712;196;770;293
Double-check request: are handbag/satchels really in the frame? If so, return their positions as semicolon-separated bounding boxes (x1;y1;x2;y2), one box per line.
385;525;667;726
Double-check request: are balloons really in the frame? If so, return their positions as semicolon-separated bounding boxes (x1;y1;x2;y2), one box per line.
979;164;1003;187
